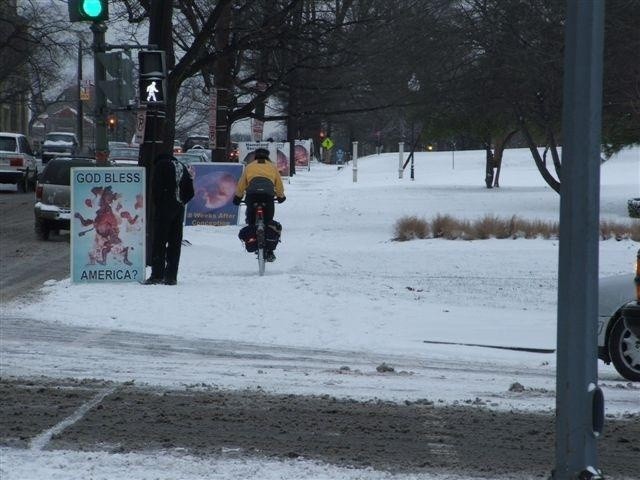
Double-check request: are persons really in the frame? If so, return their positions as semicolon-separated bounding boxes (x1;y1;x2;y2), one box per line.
233;149;285;263
144;147;195;285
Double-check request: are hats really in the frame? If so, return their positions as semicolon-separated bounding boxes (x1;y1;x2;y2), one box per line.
255;148;269;158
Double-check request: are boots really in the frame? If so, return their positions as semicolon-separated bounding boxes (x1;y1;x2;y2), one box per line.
141;272;177;285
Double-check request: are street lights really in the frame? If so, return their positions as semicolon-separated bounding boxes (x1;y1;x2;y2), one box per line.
408;70;420;179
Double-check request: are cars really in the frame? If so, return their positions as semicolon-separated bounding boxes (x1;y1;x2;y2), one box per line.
0;132;239;240
597;273;640;382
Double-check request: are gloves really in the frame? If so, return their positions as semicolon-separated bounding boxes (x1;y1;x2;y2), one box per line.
277;196;286;203
233;195;241;205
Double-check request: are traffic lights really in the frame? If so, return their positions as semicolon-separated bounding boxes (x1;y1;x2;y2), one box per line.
68;0;109;22
99;49;169;108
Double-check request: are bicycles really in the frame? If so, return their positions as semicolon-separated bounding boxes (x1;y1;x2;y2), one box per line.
234;197;285;276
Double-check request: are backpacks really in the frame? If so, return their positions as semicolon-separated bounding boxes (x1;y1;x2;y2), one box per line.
178;161;194;204
